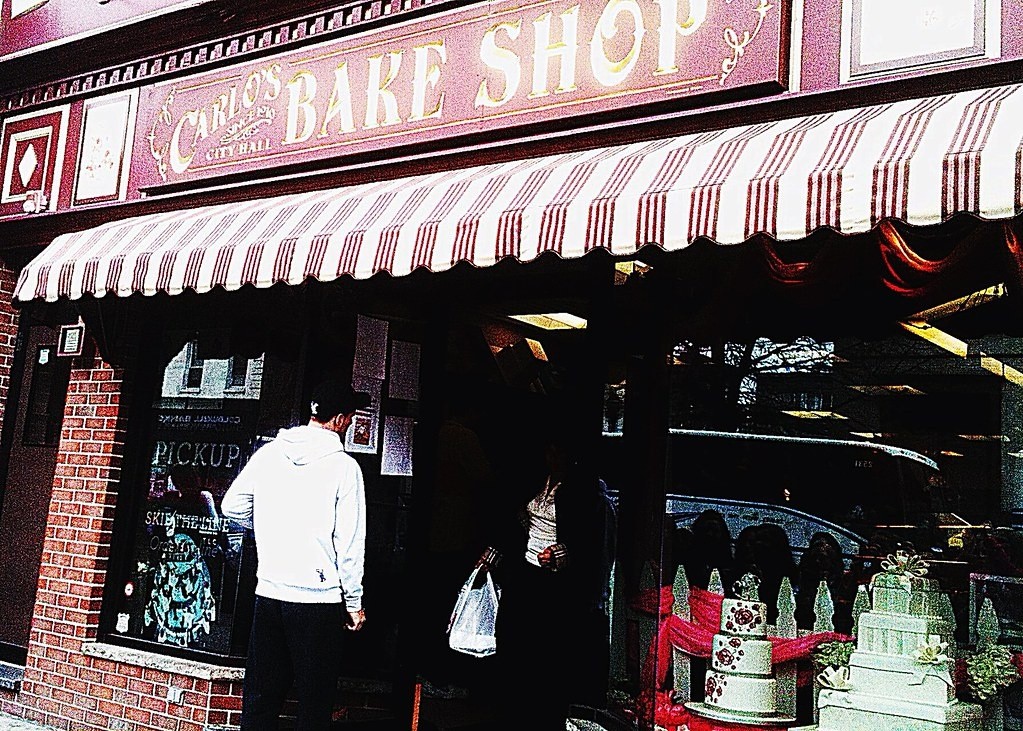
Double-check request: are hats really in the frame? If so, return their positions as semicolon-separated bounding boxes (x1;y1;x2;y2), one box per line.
311;380;371;422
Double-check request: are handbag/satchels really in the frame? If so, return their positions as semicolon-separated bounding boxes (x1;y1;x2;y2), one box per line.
446;563;503;658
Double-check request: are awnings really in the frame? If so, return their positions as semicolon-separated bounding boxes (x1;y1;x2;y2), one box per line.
11;81;1022;307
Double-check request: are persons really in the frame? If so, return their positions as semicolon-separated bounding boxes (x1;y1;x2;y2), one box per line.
472;432;617;731
220;382;368;731
